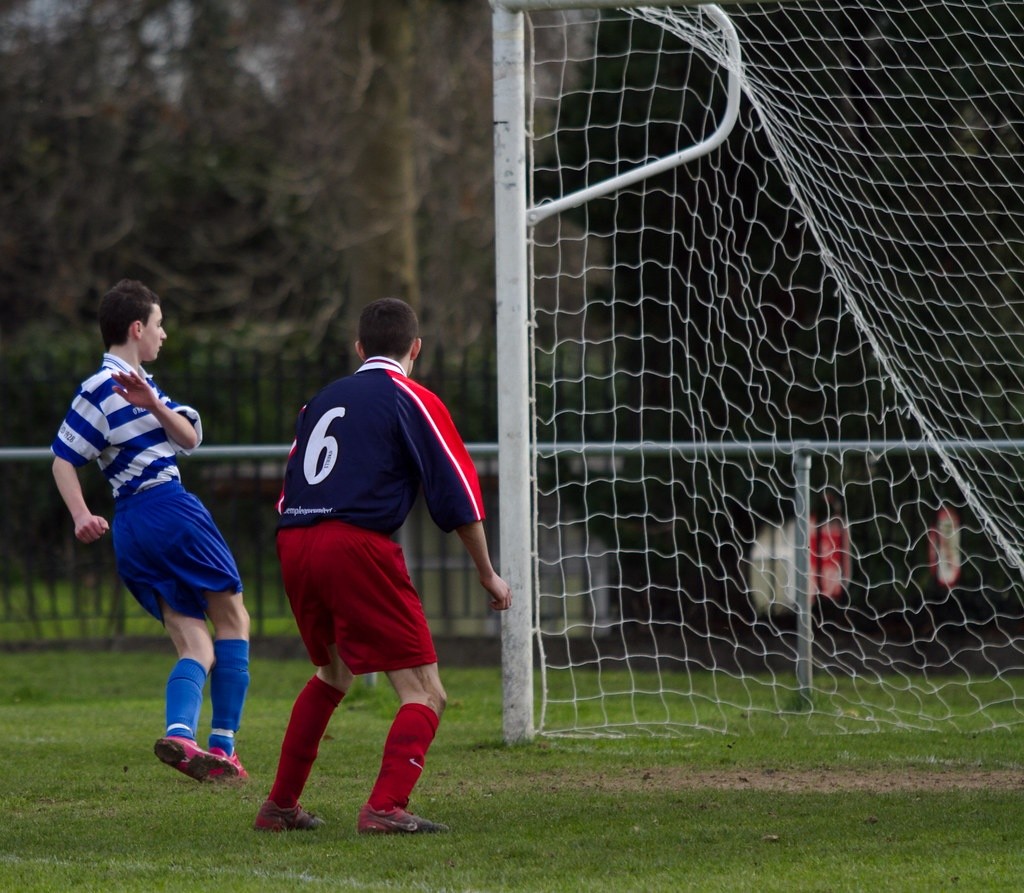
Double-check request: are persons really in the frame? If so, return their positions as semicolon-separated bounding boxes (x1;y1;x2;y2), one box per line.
254;296;515;836
50;277;257;790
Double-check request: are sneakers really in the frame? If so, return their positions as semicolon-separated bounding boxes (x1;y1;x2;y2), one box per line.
209;747;248;778
359;803;450;834
154;736;239;784
256;800;326;831
